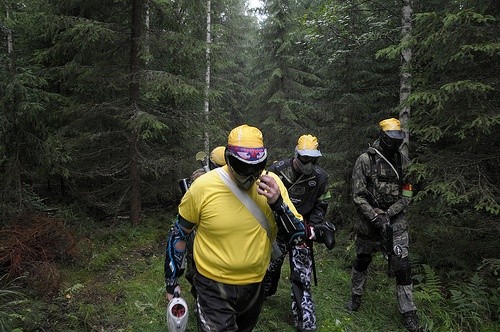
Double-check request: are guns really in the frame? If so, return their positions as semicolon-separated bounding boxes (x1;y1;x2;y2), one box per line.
380;224;393;275
299;219;335;287
167;292;189;332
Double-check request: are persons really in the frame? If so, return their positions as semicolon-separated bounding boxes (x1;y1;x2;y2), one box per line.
346;118;430;332
164;125;336;332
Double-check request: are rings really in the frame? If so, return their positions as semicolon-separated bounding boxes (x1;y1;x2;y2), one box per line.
267;187;271;192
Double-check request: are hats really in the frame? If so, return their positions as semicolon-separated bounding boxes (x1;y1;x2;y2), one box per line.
379;118;406;139
296;134;321;156
227;124;267;164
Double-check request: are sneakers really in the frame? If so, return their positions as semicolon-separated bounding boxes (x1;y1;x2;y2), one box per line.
401;312;422;332
349;294;363;311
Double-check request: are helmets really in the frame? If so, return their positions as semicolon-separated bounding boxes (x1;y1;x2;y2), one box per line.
210;146;225;167
